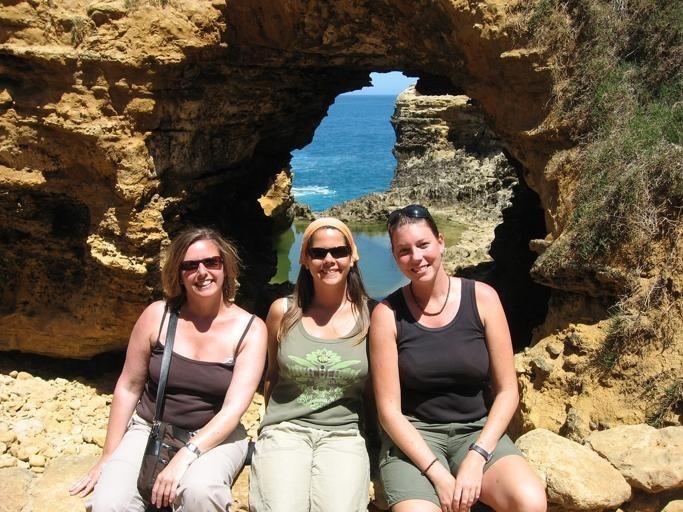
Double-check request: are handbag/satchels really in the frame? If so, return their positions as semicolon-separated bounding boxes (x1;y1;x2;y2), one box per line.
134;420;194;511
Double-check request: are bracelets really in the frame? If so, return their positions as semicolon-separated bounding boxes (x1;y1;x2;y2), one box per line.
420;457;439;475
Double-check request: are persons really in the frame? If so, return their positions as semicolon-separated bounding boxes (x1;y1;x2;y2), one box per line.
246;214;380;510
368;204;550;512
65;225;270;511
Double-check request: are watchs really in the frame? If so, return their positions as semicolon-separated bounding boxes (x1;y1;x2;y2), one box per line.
181;441;200;456
467;442;494;463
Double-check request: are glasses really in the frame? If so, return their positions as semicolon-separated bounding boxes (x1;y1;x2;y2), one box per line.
176;254;224;274
306;245;352;260
387;204;430;225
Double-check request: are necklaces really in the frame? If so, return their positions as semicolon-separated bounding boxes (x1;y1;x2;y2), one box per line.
410;273;451;316
